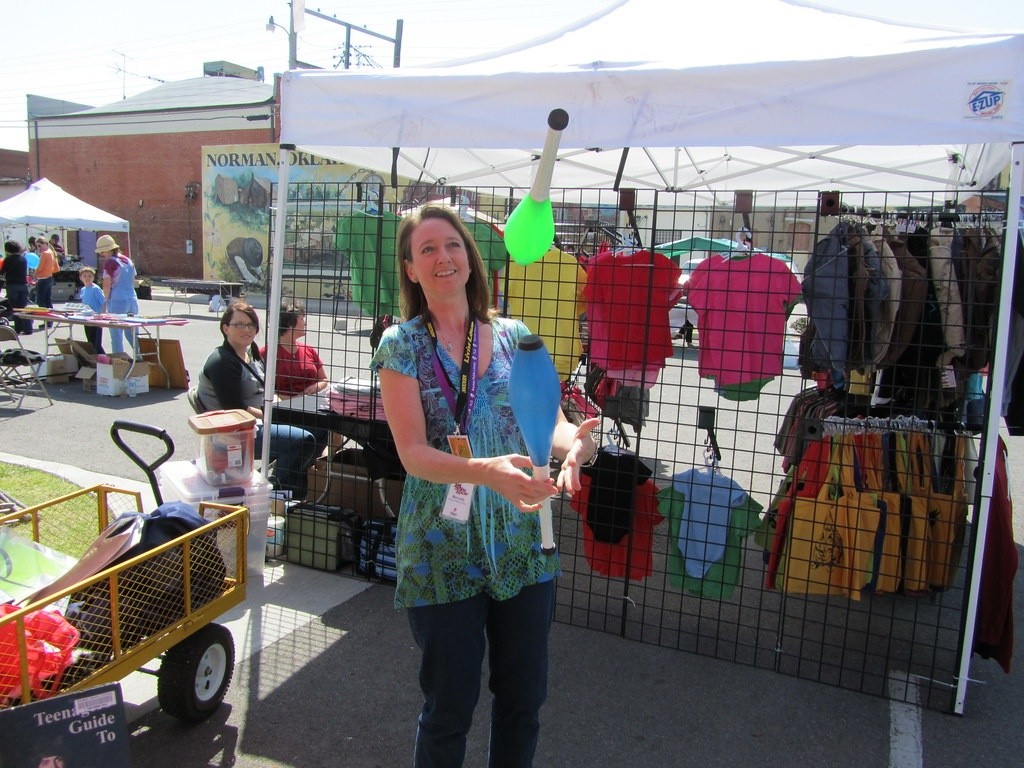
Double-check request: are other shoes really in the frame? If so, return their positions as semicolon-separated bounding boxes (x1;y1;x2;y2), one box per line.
22;331;32;334
39;323;52;330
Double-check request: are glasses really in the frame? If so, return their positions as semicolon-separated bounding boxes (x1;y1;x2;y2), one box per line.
37;244;42;247
227;322;258;330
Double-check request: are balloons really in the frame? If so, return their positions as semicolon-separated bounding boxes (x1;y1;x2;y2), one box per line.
27;253;39;268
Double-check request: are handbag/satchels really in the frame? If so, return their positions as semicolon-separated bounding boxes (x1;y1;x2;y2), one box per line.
0;603;80;704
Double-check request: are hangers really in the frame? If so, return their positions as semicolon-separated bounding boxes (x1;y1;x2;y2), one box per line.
353;173;1004;475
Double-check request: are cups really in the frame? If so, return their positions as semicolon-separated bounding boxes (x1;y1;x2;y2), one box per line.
266;516;285;557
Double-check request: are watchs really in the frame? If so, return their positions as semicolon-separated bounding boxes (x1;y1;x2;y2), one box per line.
580;442;598;468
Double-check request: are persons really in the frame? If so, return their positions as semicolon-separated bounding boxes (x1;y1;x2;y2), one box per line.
95;237;142;360
0;242;33;334
28;237;38;254
78;269;106;354
198;303;328;501
260;303;343;458
50;235;65;266
34;237;60;329
368;207;600;768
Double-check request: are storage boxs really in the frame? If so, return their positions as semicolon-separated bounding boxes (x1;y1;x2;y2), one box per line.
51;281;76;302
43;354;155;398
186;409;398;583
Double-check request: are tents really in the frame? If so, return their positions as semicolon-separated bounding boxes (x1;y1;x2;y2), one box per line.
251;36;1023;715
0;179;131;259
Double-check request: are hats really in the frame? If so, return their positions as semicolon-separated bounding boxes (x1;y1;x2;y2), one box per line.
51;234;60;242
94;235;120;252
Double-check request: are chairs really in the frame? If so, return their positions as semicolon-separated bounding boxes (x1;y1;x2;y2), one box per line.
0;325;53;410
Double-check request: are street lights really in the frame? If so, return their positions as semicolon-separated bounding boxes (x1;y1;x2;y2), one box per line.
267;16;299;72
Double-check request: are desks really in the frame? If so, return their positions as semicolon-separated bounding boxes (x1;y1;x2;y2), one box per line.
14;312;187;396
264;395;398;524
163;279;244;319
